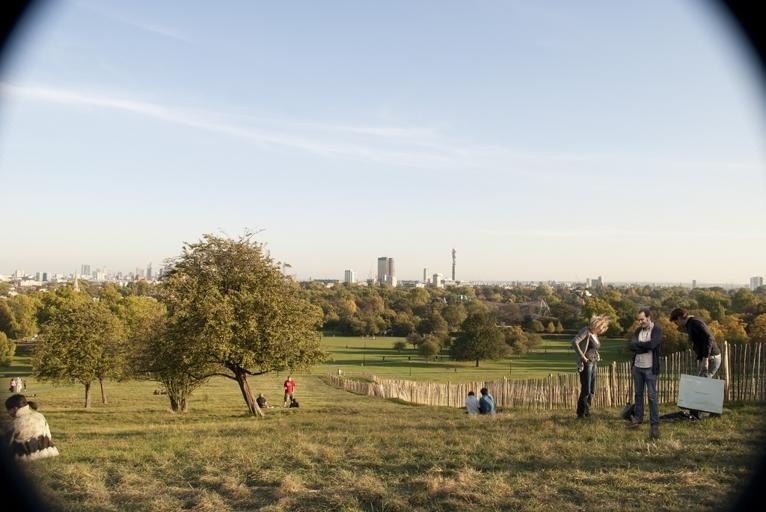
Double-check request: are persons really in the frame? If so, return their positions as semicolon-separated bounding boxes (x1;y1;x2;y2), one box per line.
464;391;480;415
479;387;495;414
9;378;16;391
624;309;663;438
282;374;296;407
669;307;724;421
571;312;611;419
4;394;59;463
256;393;273;409
15;375;22;392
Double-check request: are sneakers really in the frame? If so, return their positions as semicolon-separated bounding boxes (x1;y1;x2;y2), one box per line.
650;425;660;437
623;421;643;428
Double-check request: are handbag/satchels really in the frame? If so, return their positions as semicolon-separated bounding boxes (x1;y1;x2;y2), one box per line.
622;403;635;419
676;374;725;414
